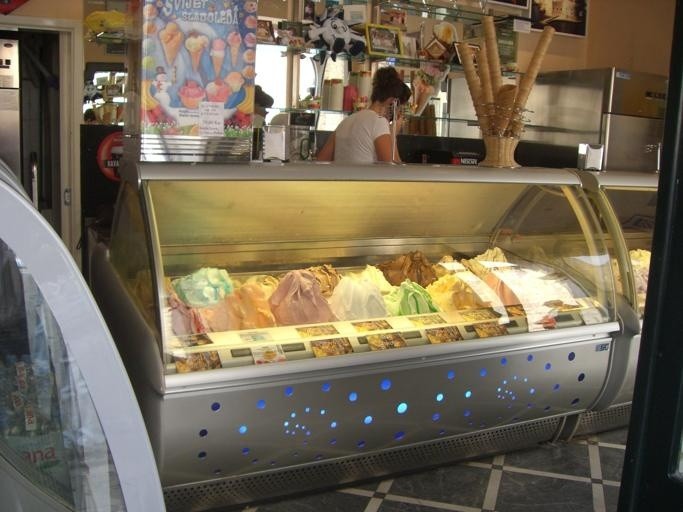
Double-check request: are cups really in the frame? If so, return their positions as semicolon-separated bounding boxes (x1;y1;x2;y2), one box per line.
455;14;556;137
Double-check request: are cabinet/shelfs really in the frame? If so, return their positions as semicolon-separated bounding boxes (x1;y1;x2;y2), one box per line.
580;168;664;510
85;159;623;512
0;163;169;512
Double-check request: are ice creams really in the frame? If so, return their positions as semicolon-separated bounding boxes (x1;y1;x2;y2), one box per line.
203;80;232;108
227;29;242;68
158;22;185;67
176;78;206;109
206;38;226;78
223;72;246;95
181;30;209;72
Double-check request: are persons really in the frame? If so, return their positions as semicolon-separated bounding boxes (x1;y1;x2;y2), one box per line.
316;66;411;164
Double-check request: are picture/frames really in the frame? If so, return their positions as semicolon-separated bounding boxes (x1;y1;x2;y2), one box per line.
454;42;480;68
425;38;447;60
365;23;404;58
